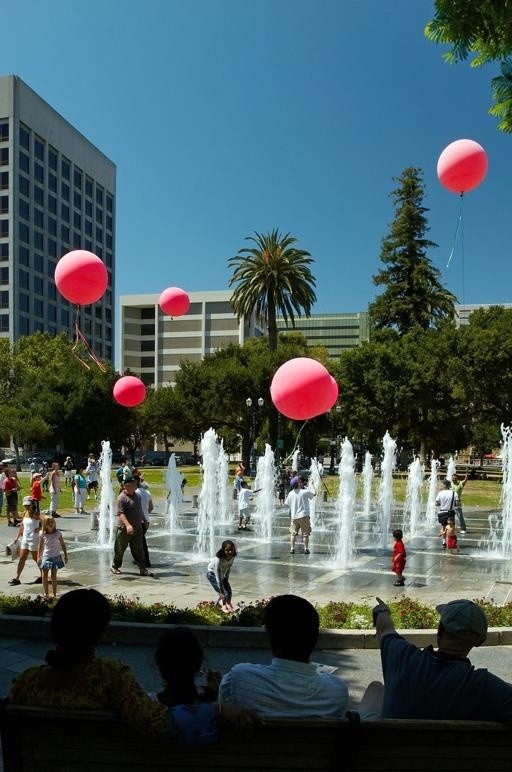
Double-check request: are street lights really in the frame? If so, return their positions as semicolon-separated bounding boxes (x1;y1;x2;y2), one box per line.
437;492;455;526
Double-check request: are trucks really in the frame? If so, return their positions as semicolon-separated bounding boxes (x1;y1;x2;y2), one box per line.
269;355;338;424
160;286;192;319
113;375;146;407
436;138;488;197
52;250;107;309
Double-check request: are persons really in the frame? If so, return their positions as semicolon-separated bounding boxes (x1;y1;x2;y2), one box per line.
234;462;247;489
438;517;460;554
450;474;468;534
36;515;68;597
6;588;176;736
87;453;95;466
86;459;98;499
64;456;73;488
133;475;154;569
116;462;125;494
2;469;19;526
8;496;42;586
32;472;50;514
278;473;287;505
206;540;238;615
10;468;22;522
40;464;49;492
111;475;156;578
387;527;410;589
71;476;75;501
358;593;512;722
284;475;317;554
73;467;87;514
218;595;348;720
49;463;62;518
131;468;140;486
435;480;459;548
29;460;39;490
0;466;6;514
235;480;262;531
150;629;216;711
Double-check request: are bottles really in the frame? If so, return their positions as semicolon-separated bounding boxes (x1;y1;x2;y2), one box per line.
32;472;42;478
124;477;137;485
435;599;488;645
290;476;299;488
22;496;32;506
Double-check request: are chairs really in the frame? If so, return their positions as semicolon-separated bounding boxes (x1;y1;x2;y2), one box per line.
110;567;120;574
227;604;234;612
34;577;42;583
442;544;446;548
222;604;229;613
304;548;310;554
402;576;406;581
8;578;20;584
140;569;153;576
290;549;295;553
393;581;404;586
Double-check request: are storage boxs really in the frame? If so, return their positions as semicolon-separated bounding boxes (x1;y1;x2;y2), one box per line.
0;708;512;772
